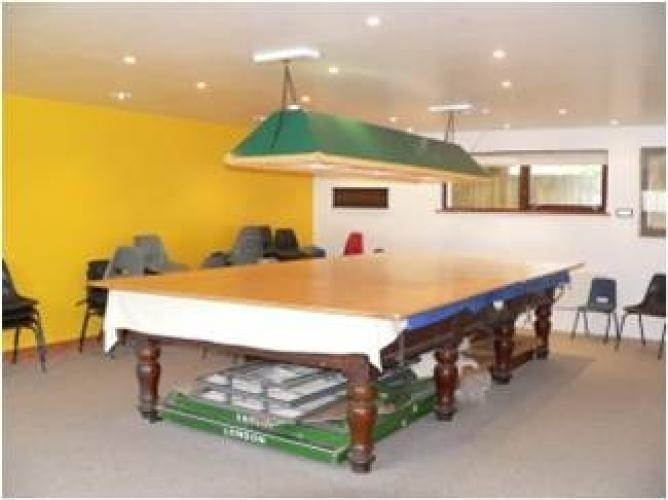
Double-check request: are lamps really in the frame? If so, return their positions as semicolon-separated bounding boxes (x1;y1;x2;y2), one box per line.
224;111;492;185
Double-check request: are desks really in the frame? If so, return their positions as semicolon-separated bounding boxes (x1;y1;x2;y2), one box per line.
85;252;586;474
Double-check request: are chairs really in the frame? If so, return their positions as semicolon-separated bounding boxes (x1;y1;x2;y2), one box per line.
2;257;47;374
78;223;326;354
614;272;666;360
570;278;621;350
